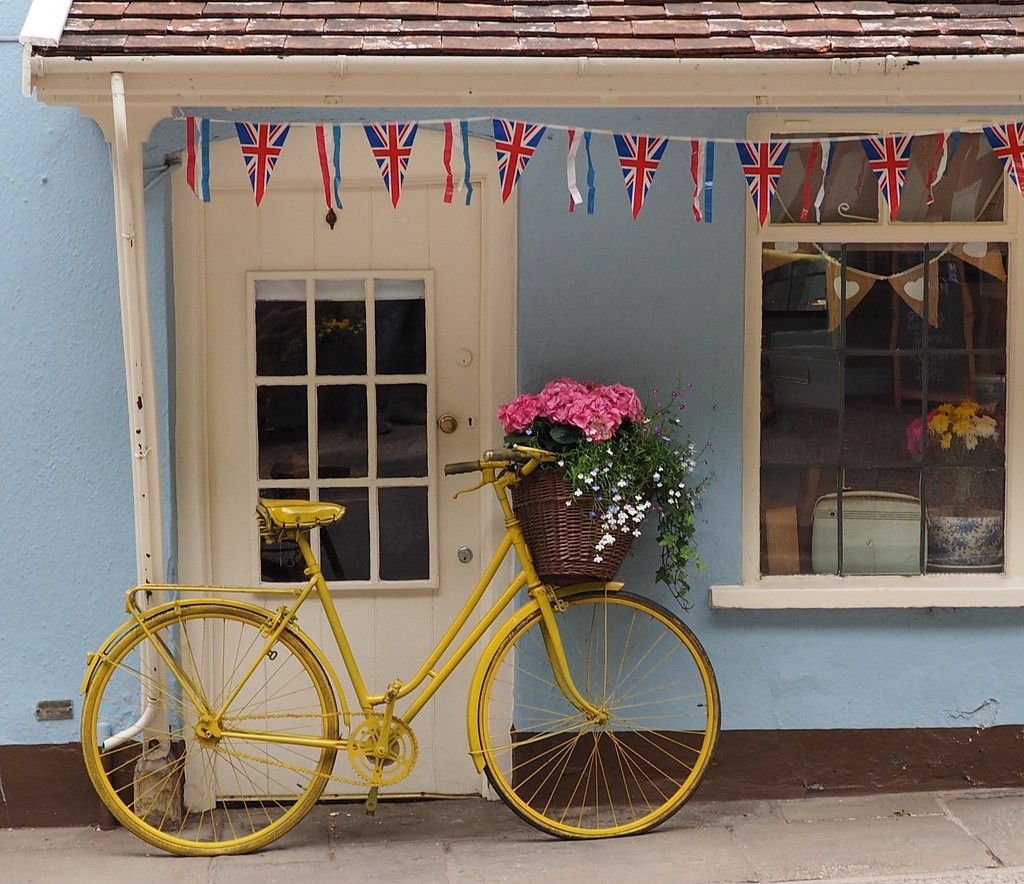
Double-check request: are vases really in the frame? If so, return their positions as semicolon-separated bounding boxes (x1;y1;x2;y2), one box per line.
927;506;1005;573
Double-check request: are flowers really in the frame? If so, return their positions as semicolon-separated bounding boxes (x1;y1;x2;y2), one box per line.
906;402;1006;516
497;373;721;614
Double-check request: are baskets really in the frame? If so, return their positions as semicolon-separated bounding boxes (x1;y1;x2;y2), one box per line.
512;467;648;584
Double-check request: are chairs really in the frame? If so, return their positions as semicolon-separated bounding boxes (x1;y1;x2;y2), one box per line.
810;491;928;577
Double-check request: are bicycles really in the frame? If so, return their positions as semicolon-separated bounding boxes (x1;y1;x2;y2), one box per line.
80;443;721;857
260;462;348;583
